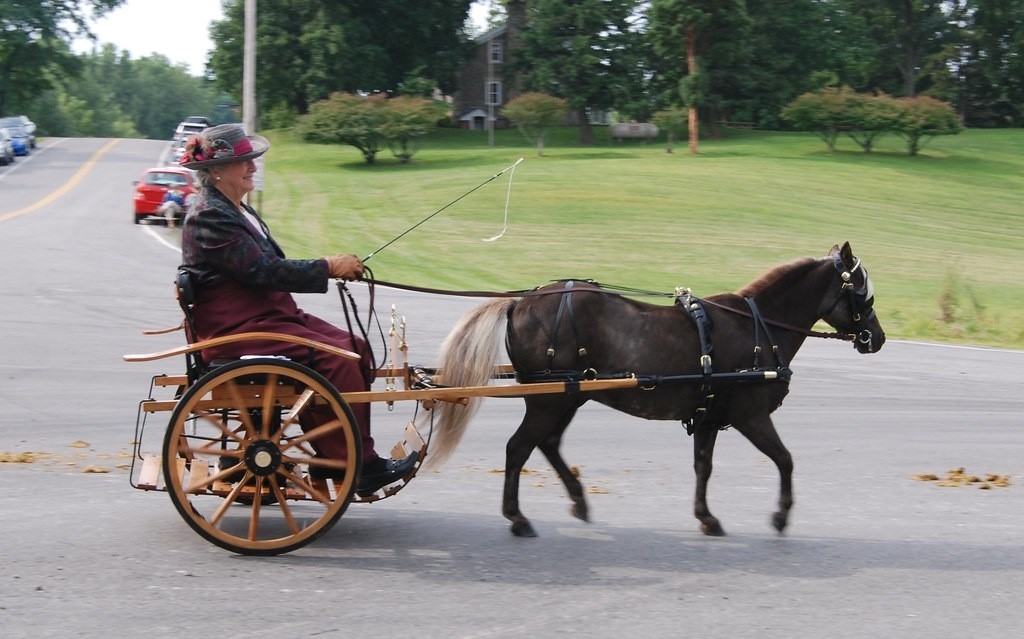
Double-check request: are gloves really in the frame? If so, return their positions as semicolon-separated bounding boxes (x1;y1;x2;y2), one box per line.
325;252;364;279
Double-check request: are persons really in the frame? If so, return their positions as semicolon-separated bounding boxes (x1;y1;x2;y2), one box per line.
155;181;201;228
181;125;418;496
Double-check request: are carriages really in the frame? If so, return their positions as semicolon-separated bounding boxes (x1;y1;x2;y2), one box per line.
121;240;887;557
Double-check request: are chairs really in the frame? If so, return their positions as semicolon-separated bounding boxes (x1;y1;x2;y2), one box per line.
175;268;294;385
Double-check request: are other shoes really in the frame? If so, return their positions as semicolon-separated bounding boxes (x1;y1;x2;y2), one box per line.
308;450;419;497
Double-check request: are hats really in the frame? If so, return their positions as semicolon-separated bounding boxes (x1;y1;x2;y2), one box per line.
178;123;270;171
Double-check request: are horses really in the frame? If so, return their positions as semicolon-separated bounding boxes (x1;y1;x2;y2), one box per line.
415;239;886;540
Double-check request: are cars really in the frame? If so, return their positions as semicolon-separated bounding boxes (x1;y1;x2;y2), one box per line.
0;115;37;149
0;126;32;157
172;122;209;141
0;128;15;165
186;116;213;128
163;166;201;189
132;168;203;225
169;139;188;162
